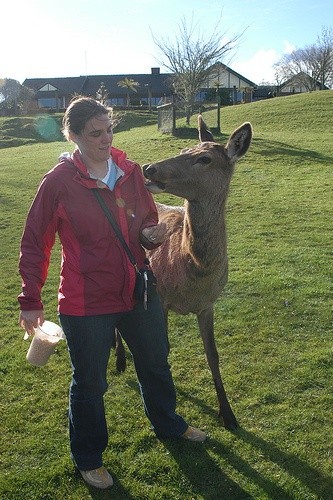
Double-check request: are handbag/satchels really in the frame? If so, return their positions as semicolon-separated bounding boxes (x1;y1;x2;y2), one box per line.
133;270;158;332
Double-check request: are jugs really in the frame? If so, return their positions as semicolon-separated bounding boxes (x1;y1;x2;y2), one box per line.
23;317;66;367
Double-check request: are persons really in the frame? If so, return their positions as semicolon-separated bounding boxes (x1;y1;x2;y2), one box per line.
18;95;206;489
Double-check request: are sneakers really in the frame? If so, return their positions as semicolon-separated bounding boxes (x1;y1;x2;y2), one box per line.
180;426;206;443
71;452;113;489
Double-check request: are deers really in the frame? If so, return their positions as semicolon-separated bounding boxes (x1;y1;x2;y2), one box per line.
112;115;250;420
